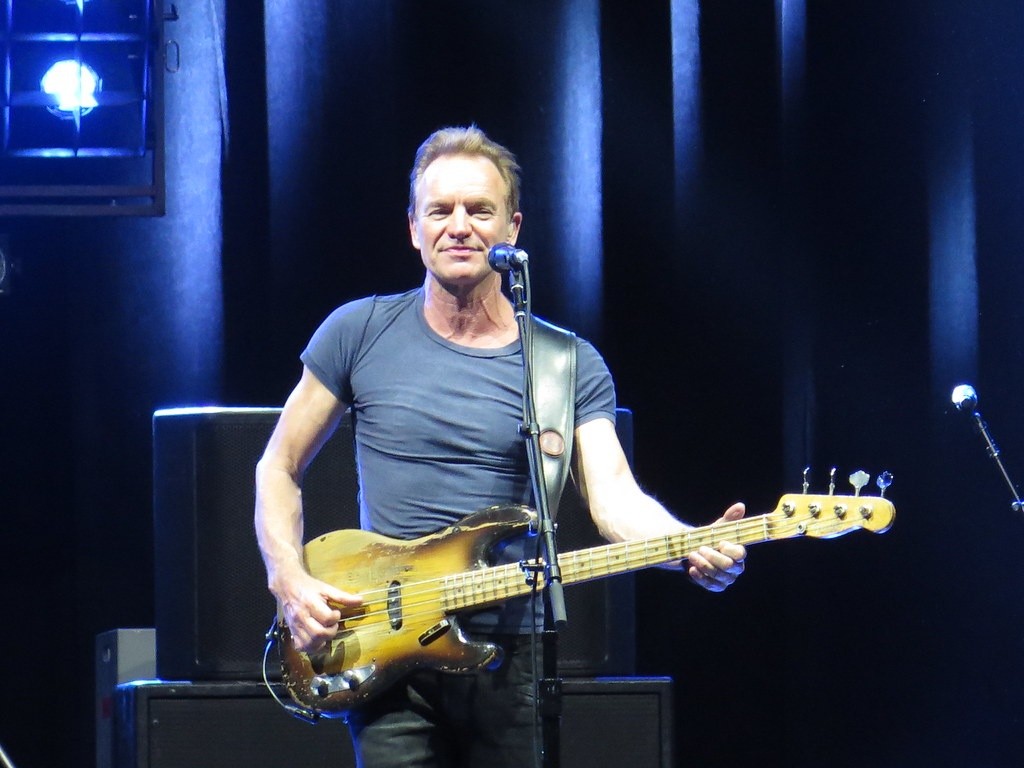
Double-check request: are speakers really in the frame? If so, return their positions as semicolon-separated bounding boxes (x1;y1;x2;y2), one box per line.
542;674;677;768
117;680;357;768
154;406;359;680
543;409;637;674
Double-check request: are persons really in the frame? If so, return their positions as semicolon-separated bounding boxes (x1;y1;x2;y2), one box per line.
250;128;749;768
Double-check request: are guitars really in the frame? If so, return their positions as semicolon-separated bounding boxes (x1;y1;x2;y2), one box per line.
260;462;899;723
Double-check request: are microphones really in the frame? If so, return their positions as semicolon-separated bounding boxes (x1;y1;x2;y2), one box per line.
487;242;529;274
952;385;978;413
